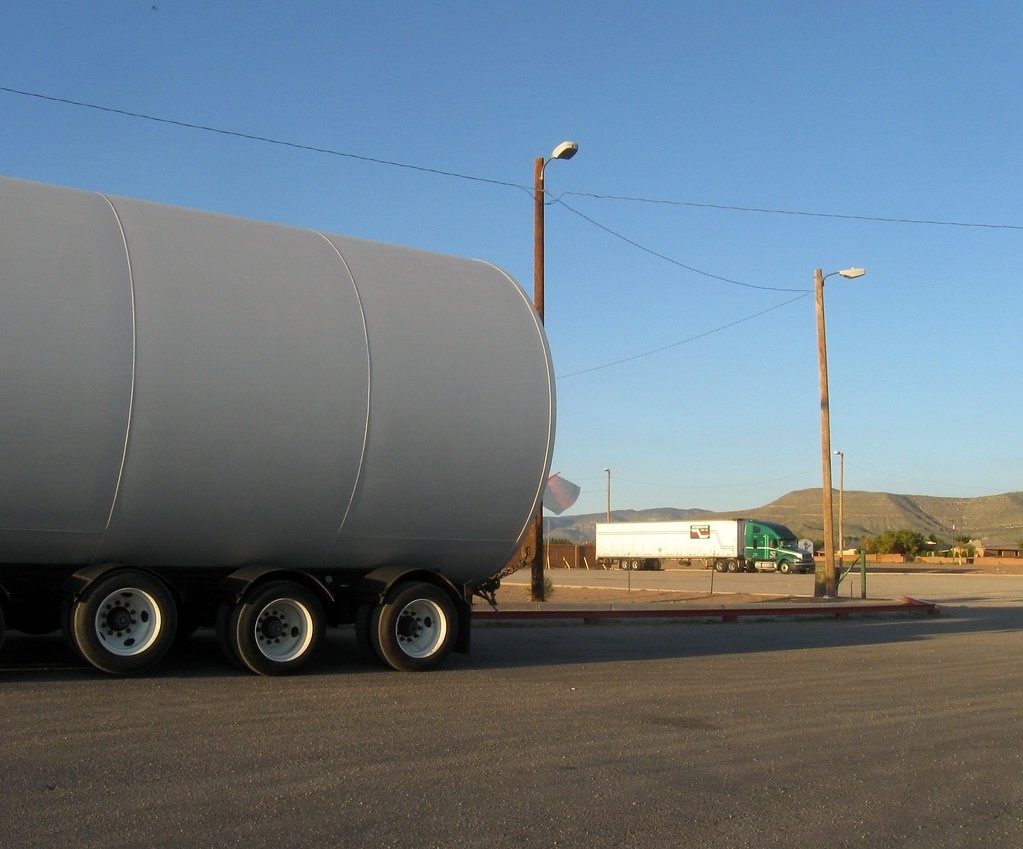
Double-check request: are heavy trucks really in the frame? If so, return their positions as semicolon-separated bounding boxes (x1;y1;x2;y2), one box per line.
593;520;816;576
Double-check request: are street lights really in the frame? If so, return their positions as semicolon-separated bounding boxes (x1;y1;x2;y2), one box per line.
812;265;865;595
531;141;581;607
604;467;612;521
832;450;844;574
